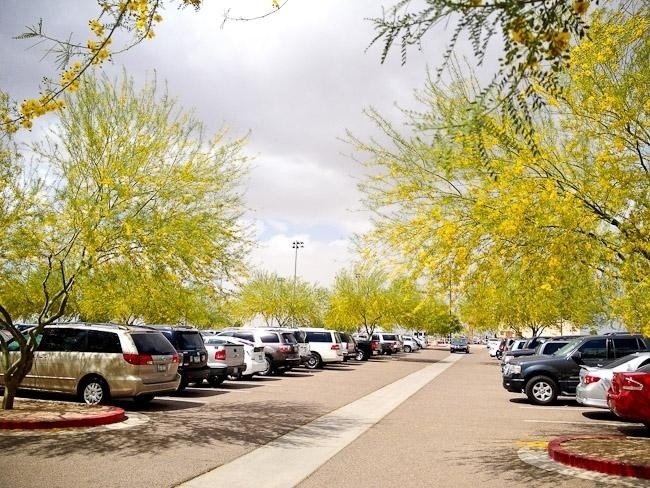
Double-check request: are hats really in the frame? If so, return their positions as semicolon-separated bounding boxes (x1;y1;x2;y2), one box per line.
473;339;481;344
483;332;650;429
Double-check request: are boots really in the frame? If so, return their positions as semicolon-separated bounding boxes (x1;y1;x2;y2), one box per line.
450;335;470;354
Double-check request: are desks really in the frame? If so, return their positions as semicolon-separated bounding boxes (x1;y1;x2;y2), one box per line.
290;238;306;325
